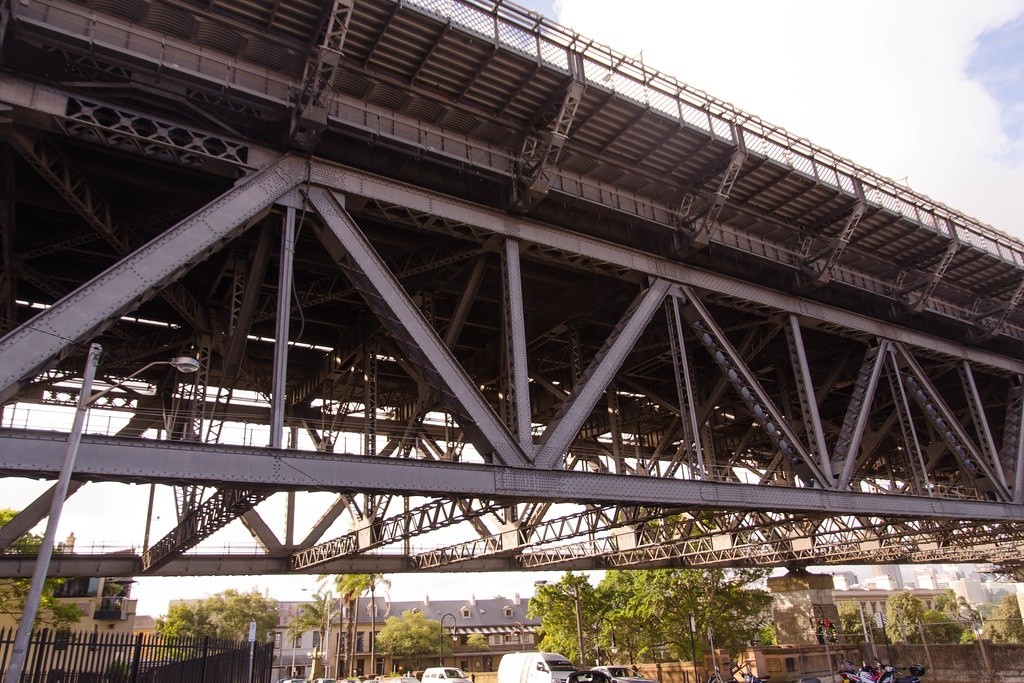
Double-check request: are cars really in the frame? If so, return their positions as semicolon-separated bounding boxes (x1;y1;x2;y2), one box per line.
565;663;658;683
277;675;422;683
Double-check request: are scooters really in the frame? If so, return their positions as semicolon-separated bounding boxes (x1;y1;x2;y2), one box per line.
837;657;927;683
705;661;822;683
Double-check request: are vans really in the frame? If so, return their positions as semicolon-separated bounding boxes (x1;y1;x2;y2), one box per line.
421;666;473;683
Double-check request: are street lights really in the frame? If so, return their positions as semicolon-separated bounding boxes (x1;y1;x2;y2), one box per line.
5;341;200;682
534;580;586;665
438;613;458;667
300;588;330;679
595;616;619;666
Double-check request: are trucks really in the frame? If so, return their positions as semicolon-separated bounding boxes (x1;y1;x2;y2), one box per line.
497;652;580;683
